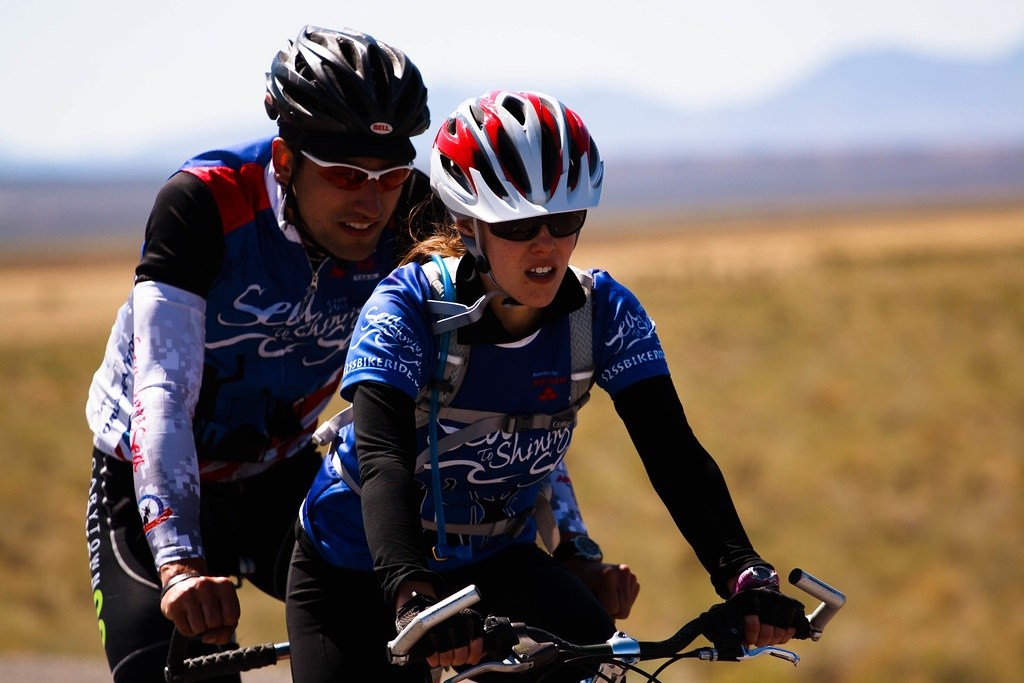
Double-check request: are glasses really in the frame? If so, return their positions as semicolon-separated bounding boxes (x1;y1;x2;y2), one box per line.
300;148;415;194
488;207;587;243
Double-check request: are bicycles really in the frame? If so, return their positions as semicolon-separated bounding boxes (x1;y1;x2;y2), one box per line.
164;553;846;683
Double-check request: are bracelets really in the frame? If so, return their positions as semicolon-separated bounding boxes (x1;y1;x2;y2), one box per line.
160;573;204;598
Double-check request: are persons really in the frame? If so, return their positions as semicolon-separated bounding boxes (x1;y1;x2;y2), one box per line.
285;91;814;683
85;25;641;683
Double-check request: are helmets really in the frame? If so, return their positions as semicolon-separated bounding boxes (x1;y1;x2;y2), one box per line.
264;25;431;142
429;89;605;223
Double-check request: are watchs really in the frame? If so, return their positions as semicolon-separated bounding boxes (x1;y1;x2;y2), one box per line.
732;566;780;596
